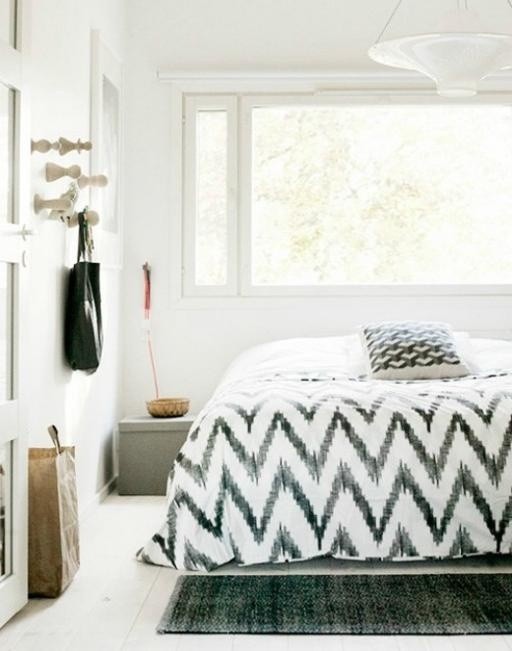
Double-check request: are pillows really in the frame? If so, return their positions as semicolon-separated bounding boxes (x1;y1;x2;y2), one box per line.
270;319;509;381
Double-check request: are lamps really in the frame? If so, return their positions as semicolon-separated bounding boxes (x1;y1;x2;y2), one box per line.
368;1;512;101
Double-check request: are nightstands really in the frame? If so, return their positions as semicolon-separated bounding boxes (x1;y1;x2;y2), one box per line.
117;415;196;495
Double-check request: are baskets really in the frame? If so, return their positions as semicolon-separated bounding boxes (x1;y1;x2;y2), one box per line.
147;397;191;418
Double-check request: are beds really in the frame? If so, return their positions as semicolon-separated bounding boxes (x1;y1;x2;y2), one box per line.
138;334;512;574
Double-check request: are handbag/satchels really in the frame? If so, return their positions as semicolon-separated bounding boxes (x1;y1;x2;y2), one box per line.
28;422;80;599
62;210;103;375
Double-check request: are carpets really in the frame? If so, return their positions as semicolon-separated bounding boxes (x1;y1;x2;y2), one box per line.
157;574;512;636
